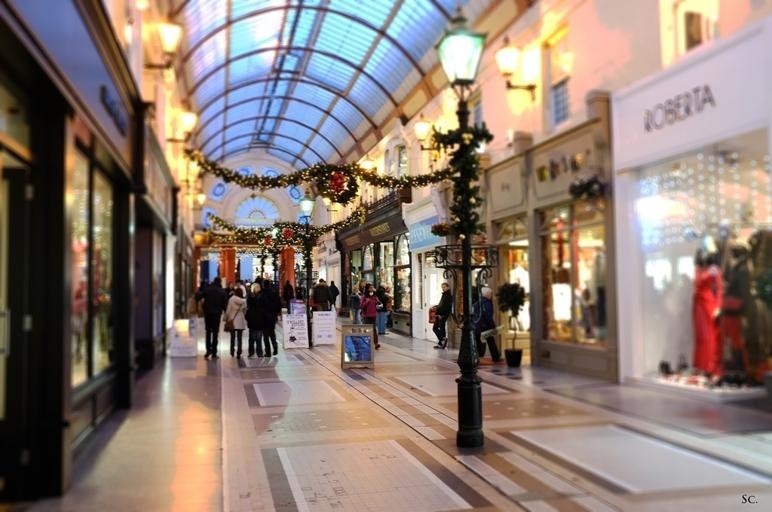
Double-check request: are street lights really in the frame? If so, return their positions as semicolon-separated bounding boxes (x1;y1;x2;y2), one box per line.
434;7;489;449
299;188;316;348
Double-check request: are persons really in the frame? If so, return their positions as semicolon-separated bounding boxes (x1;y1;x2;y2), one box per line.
690;239;763;391
193;274;501;364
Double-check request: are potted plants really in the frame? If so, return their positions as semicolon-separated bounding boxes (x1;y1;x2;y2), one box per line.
497;281;525;368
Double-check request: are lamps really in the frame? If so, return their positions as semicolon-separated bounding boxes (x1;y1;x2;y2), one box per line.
361;149;376;174
165;100;202;144
495;34;536;91
413;112;442;153
323;194;340;215
143;20;183;73
192;188;207;214
